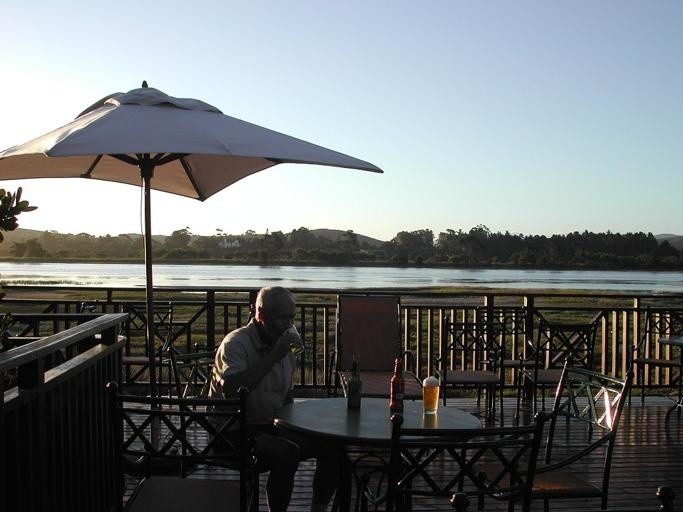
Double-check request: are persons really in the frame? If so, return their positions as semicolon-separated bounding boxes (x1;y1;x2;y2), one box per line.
210;287;342;512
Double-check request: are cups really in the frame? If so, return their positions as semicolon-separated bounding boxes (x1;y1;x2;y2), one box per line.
282;323;306;355
422;384;439;416
423;416;440;429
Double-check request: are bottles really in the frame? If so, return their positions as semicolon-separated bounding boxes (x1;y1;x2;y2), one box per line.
345;354;363;419
388;357;406;416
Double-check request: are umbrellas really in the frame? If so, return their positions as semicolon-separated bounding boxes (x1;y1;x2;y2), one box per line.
0;79;383;449
